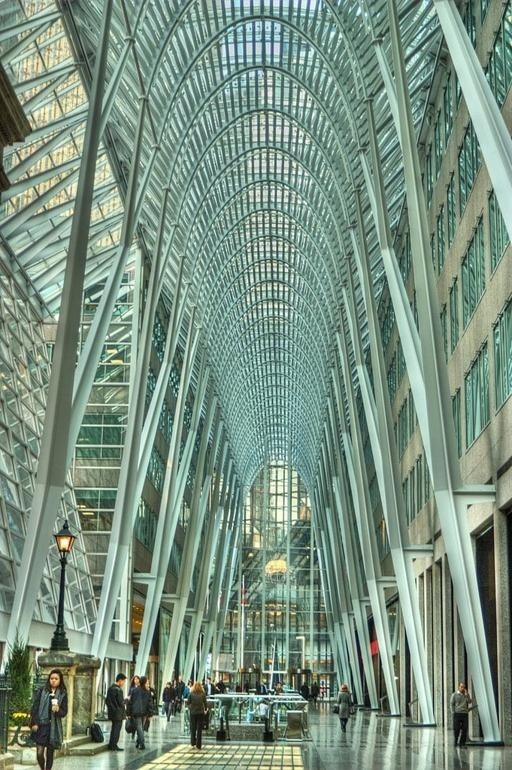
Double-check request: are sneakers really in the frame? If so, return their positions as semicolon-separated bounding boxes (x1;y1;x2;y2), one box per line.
135;740;146;749
108;744;125;751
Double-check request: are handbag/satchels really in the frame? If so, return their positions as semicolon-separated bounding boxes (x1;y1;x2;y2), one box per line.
333;704;339;713
125;719;134;734
349;704;355;714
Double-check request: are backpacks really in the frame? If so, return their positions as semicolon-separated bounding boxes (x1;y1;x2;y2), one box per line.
91;722;104;743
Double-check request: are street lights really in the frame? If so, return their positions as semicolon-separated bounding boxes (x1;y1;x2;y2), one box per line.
50;520;76;651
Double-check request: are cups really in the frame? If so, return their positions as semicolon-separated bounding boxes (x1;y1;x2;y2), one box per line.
51;699;58;713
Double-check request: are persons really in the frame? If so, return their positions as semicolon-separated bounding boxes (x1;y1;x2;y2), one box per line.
450;681;472;749
337;684;354;733
105;673;320;751
28;669;67;770
